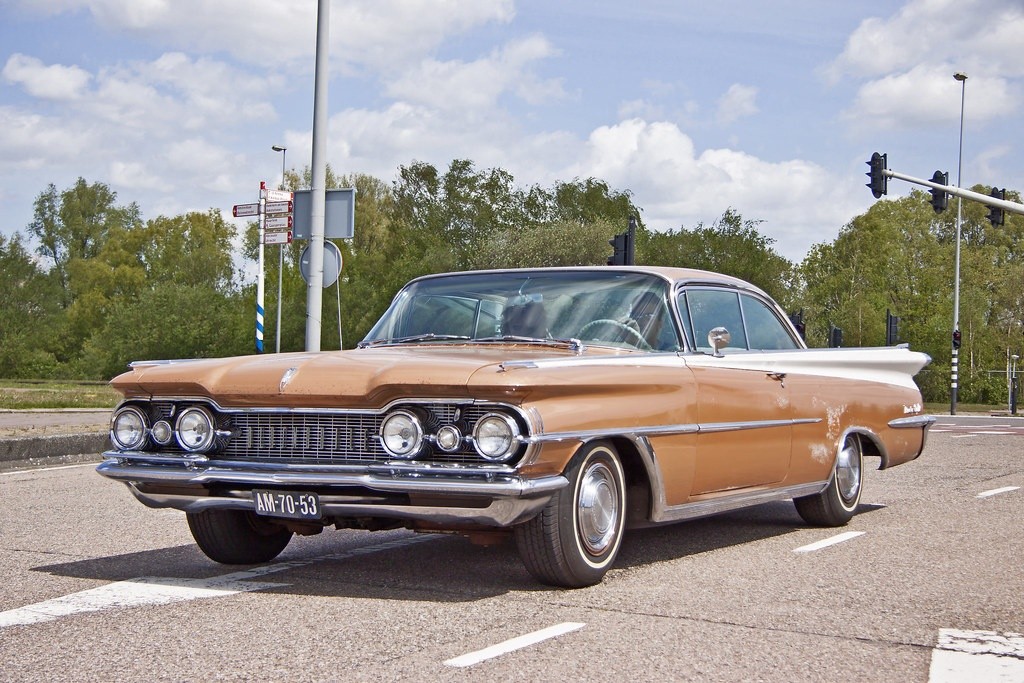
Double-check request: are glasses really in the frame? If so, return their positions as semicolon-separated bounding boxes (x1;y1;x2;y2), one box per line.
632;313;653;319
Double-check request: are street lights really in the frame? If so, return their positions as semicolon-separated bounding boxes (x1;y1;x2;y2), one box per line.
948;70;968;414
271;144;287;352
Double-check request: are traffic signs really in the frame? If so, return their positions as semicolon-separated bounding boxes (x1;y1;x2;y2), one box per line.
266;189;294;203
265;231;293;245
265;216;293;229
265;201;293;214
232;203;260;218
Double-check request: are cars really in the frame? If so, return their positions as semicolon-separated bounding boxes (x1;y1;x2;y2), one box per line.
94;262;935;590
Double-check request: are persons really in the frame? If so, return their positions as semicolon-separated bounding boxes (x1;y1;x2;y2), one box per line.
613;292;676;351
496;289;549;338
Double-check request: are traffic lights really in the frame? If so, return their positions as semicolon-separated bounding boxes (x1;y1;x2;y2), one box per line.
983;186;1001;229
865;152;883;199
953;330;962;347
834;327;843;346
927;171;945;214
891;315;900;343
606;233;627;266
